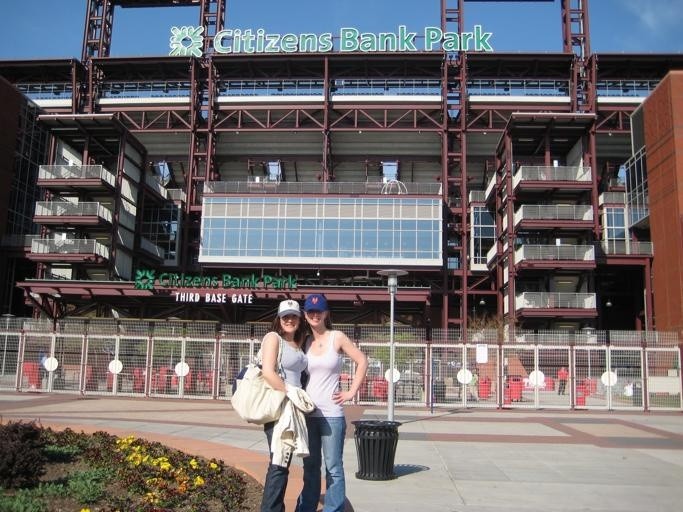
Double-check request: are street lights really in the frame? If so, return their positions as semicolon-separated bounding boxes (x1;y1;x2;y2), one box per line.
376;265;408;440
0;312;15;377
581;325;598;387
167;315;182;372
219;327;227;370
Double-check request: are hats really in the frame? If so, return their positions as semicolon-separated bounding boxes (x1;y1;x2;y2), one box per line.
304;294;328;312
277;299;301;318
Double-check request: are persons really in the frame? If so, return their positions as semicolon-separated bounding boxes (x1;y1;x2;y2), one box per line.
469;369;480;402
558;367;569;395
254;299;315;512
294;293;369;512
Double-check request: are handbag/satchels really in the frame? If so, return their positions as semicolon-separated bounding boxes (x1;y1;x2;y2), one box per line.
230;363;286;425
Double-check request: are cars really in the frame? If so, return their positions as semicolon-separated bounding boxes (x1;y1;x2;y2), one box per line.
188;351;478;386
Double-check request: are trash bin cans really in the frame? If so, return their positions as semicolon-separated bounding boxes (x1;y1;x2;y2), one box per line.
633;382;643;407
475;372;599;408
23;362;221;394
351;421;402;481
339;374;393;411
